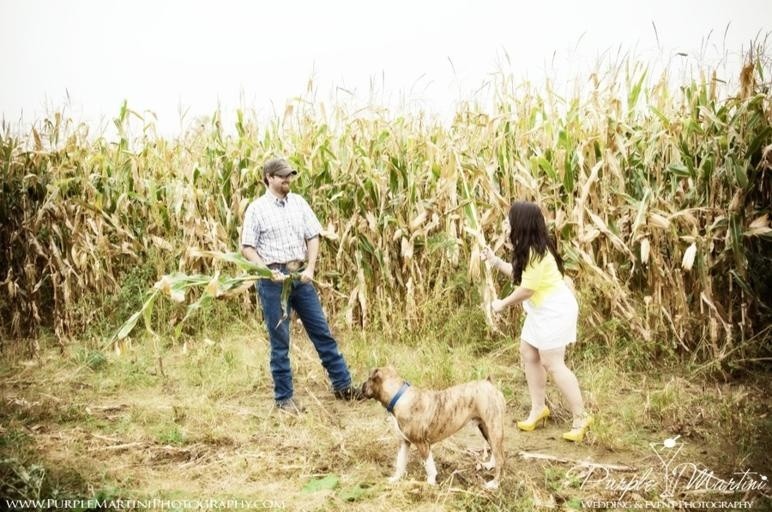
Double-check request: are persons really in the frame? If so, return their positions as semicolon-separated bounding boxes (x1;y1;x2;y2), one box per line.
242;160;368;410
483;201;594;442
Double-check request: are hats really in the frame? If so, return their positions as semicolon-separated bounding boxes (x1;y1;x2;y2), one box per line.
263;158;299;176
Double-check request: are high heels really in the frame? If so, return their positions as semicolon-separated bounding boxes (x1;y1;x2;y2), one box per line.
517;408;551;433
563;415;593;442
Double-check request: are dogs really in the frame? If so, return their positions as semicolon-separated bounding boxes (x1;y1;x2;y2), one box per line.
362;362;508;488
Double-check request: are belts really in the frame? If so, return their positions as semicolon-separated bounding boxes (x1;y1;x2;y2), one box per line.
268;262;303;272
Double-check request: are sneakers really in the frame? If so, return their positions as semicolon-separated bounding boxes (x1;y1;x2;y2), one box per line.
335;384;365;402
